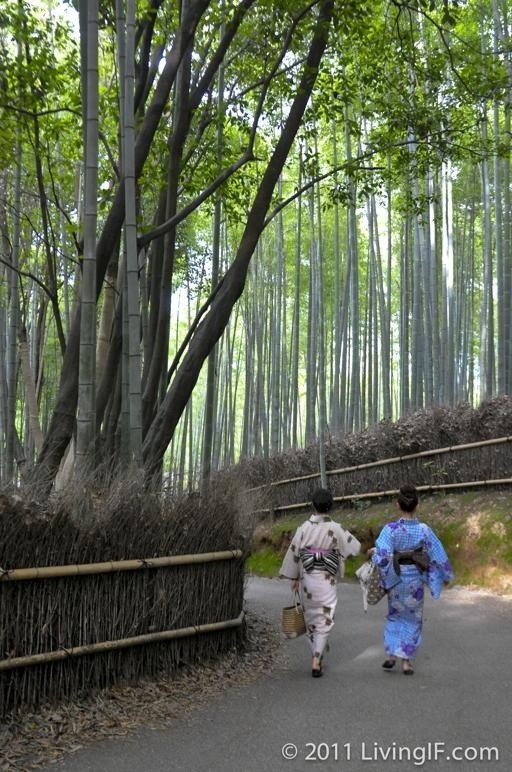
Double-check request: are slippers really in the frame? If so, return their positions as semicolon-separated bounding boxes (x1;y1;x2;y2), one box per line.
404;664;414;675
312;665;321;678
383;660;396;668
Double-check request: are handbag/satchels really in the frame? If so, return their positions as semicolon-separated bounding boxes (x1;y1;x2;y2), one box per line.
283;603;306;640
367;564;387;605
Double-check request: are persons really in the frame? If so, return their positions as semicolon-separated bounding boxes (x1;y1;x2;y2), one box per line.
277;487;361;679
364;485;455;676
